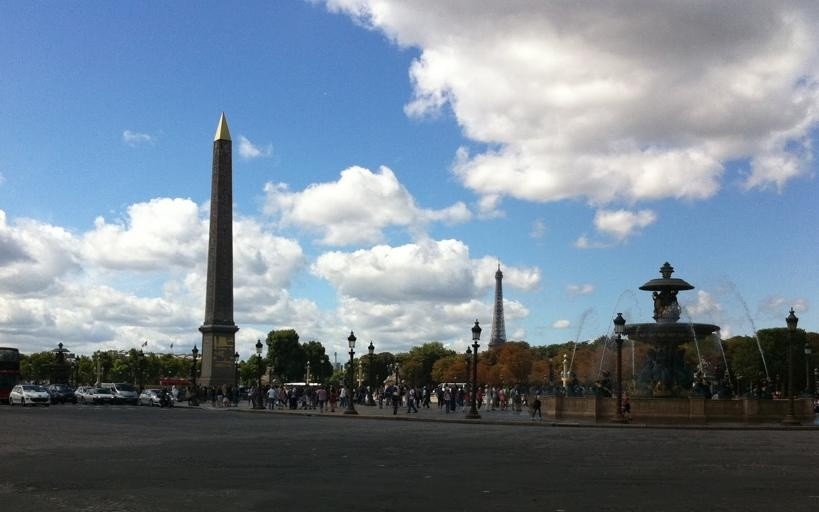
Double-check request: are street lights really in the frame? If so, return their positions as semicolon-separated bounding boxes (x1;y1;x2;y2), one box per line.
463;319;483;418
254;338;266;409
803;341;814;394
611;310;630;424
464;344;472;408
782;306;803;428
189;343;199;407
775;373;780;401
365;340;378;407
233;351;241;408
342;331;359;414
385;356;401;387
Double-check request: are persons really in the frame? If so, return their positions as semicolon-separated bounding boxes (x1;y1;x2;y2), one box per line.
378;381;542;420
157;383;366;413
621;391;632;423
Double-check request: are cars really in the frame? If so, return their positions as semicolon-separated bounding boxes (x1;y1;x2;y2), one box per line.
8;382;179;406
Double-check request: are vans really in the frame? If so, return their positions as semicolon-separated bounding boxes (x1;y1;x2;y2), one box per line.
432;381;467;395
